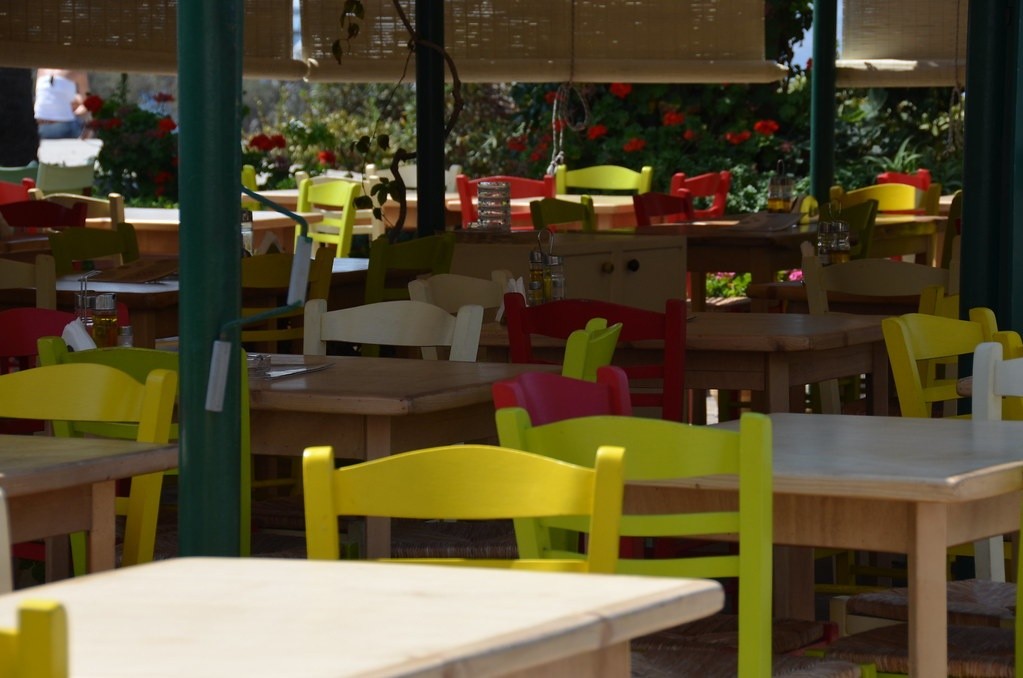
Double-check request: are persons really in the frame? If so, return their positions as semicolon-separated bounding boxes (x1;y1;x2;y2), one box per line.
33;68;84;139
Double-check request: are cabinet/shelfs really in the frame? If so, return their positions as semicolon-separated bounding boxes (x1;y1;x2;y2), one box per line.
453;232;687;307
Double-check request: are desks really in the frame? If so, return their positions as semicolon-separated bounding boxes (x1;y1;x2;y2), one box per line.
0;435;178;591
638;213;951;425
170;351;563;559
748;257;950;413
382;194;634;230
240;187;298;210
84;208;323;257
32;272;179;327
477;312;892;413
0;555;726;678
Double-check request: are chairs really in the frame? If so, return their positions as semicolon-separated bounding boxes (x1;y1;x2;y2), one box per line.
348;317;623;560
240;247;337;340
303;298;485;362
555;164;652;233
0;363;178;565
38;334;251;576
800;241;951;415
239;164;388;256
829;182;942;267
944;189;964;269
302;443;627;574
493;366;838;655
502;293;712;557
408;268;511;361
634;190;696;299
876;169;931;262
497;408;878;678
664;170;730;224
825;306;1023;678
457;173;555;232
0;161;139;373
530;194;597;233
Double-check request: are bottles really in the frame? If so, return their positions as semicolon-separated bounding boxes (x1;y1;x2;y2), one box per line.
525;249;568;307
74;291;134;348
814;218;850;266
